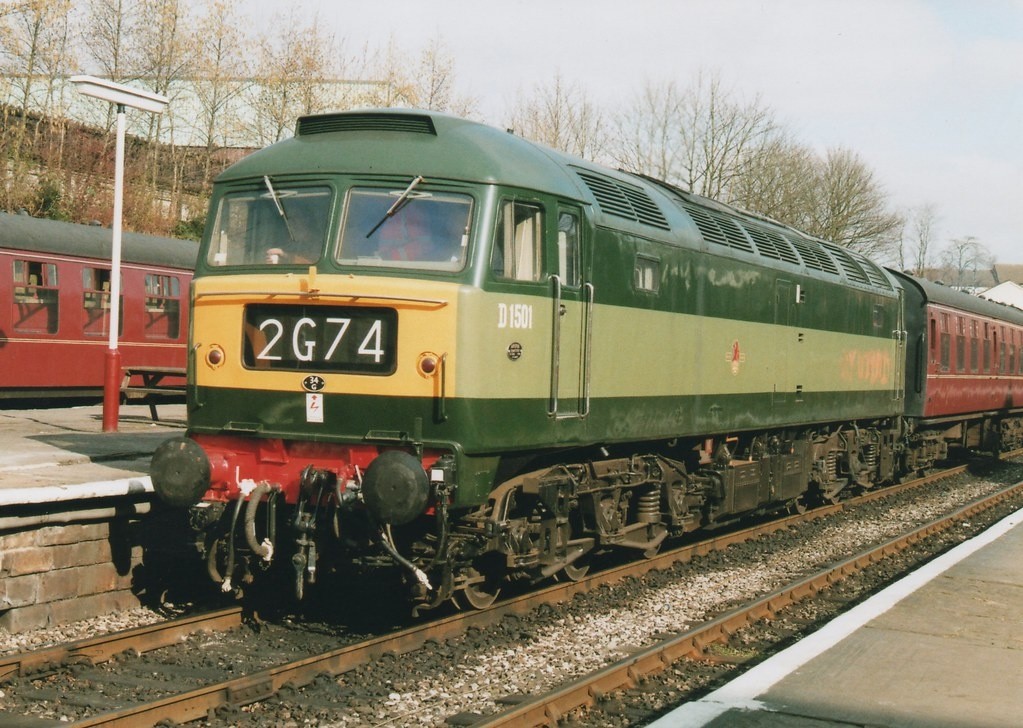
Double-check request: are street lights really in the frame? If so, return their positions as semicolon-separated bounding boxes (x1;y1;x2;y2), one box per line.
67;74;169;432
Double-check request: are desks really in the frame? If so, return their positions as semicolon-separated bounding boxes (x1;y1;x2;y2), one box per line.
121;366;186;419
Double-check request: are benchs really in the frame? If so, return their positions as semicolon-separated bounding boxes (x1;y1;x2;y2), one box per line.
120;387;187;421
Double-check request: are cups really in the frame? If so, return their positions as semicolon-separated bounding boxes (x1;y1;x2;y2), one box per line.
270;254;279;264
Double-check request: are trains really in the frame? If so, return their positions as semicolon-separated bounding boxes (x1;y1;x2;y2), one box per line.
147;107;1023;617
0;210;197;405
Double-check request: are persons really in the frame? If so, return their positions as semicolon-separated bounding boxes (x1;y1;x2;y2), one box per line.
265;202;321;264
446;211;504;275
